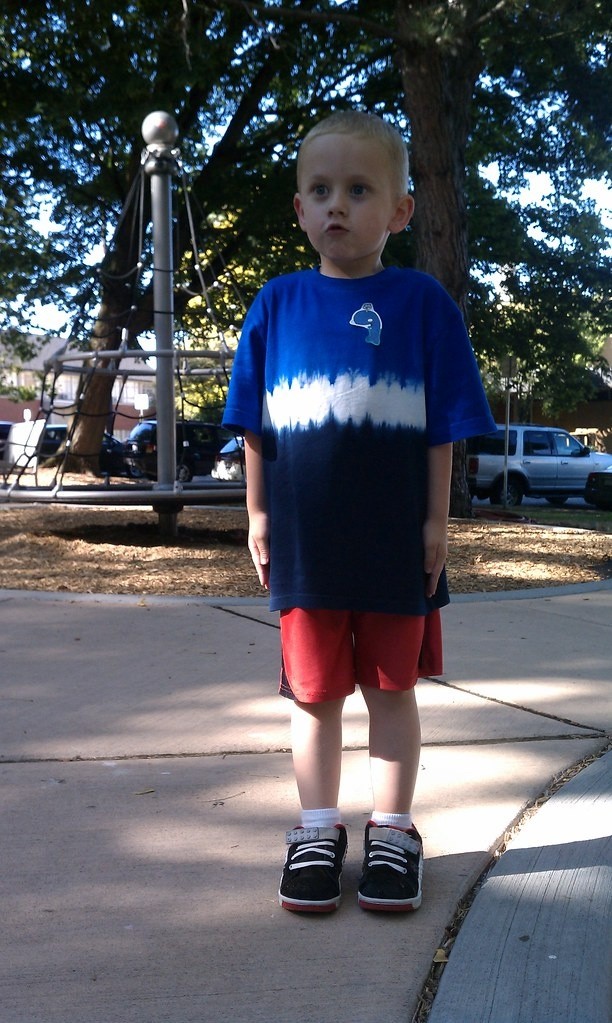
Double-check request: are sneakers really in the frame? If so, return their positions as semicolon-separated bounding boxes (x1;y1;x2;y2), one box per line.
356;821;423;911
278;823;348;912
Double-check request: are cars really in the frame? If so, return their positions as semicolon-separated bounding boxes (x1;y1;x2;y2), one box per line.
0;420;14;459
212;435;245;482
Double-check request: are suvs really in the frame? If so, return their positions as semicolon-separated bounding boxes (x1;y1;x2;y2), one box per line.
37;424;127;475
463;423;612;505
124;419;240;482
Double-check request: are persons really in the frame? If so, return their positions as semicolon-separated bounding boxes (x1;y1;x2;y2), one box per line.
223;111;498;914
